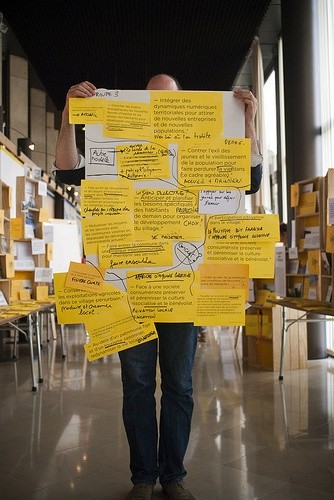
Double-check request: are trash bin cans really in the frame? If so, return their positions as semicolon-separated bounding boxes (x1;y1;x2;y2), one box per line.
307;313;328;360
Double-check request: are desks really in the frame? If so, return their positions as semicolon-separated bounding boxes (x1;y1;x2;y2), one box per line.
266;296;334;383
0;296;89;393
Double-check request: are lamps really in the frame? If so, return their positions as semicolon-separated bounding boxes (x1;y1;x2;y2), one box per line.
65;186;79;203
16;137;36;156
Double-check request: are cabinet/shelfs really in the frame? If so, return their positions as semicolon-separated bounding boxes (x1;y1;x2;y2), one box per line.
287;176;322;297
322;168;334;255
17;175;43;241
0;179;15;306
14;239;42;297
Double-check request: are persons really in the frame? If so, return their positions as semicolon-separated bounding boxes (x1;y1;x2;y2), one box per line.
51;73;263;500
